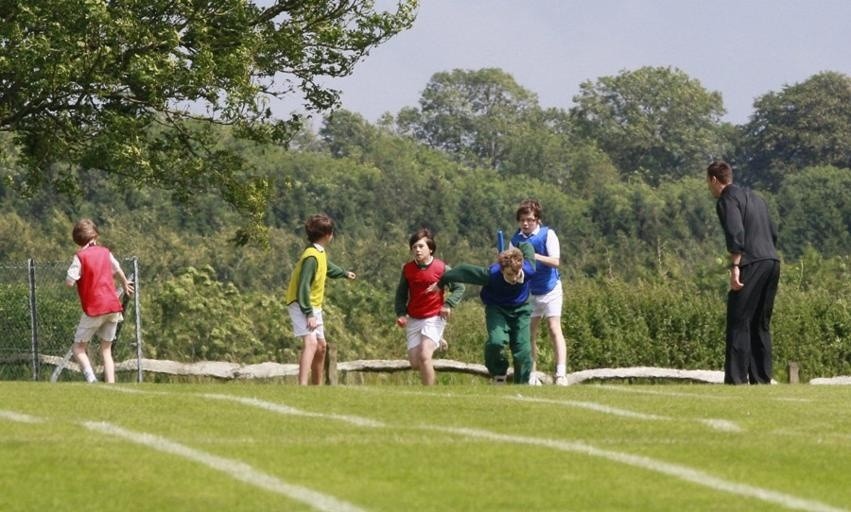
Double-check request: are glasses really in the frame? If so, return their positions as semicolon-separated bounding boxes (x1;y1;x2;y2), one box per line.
519;218;537;222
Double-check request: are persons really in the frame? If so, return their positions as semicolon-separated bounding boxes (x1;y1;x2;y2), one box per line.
62;215;137;385
392;228;466;390
507;198;569;384
704;162;785;385
283;212;358;388
424;238;538;385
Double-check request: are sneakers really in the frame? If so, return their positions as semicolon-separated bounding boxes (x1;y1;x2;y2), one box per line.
555;375;567;386
529;377;541;386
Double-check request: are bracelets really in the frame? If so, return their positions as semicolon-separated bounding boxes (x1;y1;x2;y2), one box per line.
730;262;742;268
